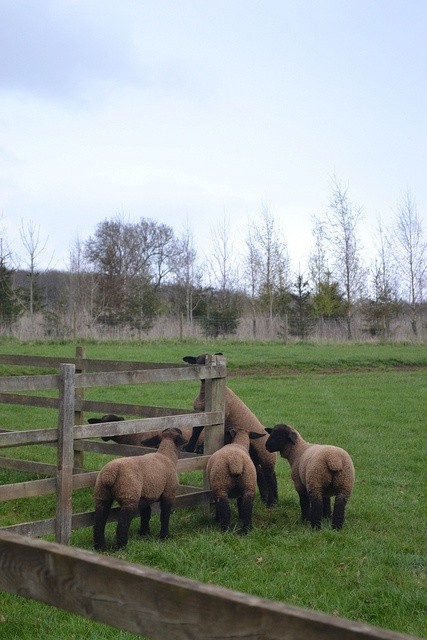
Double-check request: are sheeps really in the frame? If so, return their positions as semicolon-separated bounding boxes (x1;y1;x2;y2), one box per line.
89;415;205;454
264;424;355;531
206;428;266;535
183;352;276;482
93;428;186;552
